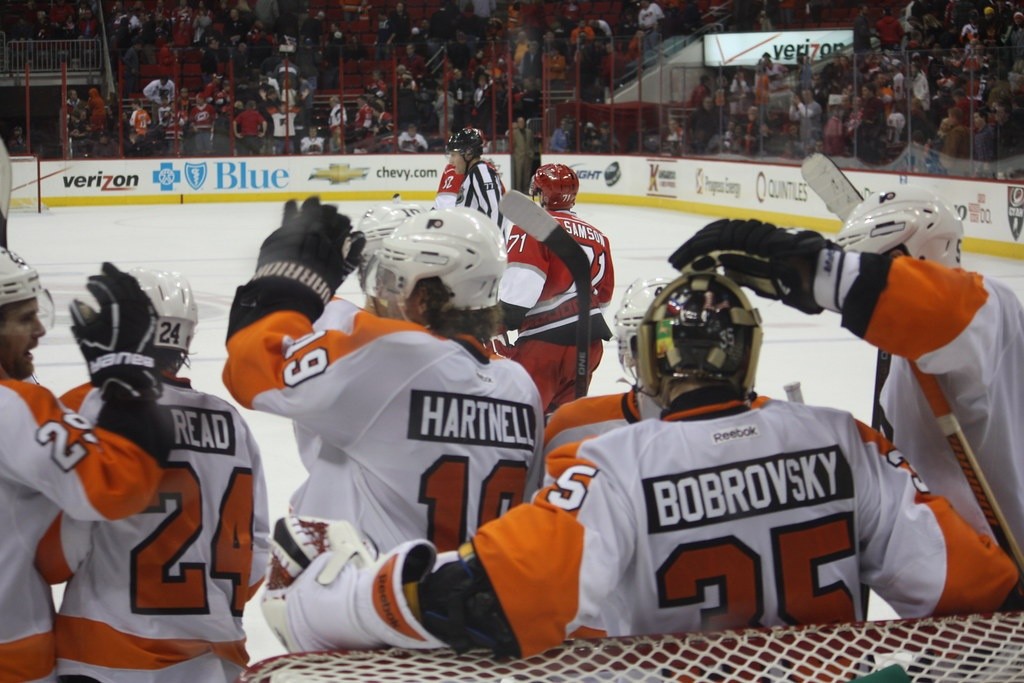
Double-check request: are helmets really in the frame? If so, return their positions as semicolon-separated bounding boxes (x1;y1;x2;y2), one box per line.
0;248;41;307
616;278;676;370
833;191;967;270
349;198;426;295
373;208;509;312
630;271;763;410
445;127;486;163
130;266;199;353
527;164;580;211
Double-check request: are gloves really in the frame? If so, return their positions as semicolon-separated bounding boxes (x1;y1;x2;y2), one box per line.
68;263;171;404
254;192;368;306
264;516;452;654
666;217;843;315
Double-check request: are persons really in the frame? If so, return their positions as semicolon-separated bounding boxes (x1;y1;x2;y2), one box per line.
434;126;508;240
1;245;178;683
667;185;1023;682
0;2;1021;183
540;274;665;488
485;163;617;429
31;267;272;681
222;191;551;552
251;270;1017;683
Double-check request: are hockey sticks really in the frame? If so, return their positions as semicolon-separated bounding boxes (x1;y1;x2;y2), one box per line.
498;190;589;396
800;152;1022;574
